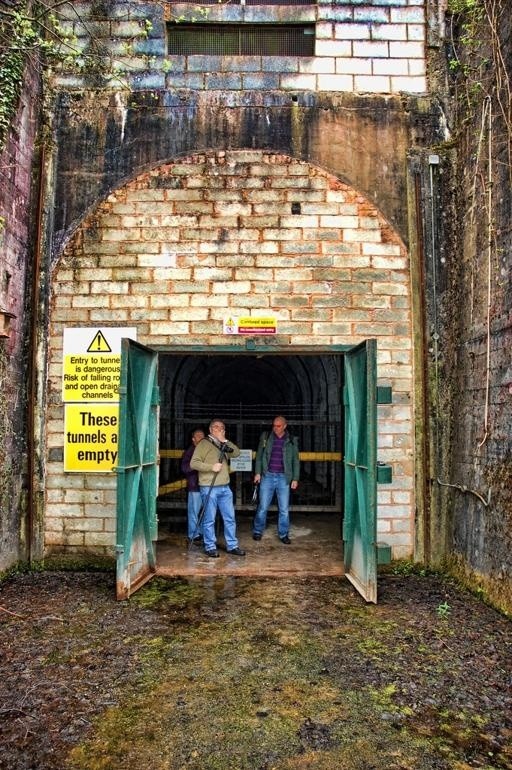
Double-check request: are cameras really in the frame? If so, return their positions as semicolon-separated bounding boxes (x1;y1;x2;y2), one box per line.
220;442;234;453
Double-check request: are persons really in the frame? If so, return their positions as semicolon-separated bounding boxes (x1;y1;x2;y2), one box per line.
190;419;245;558
180;428;217;546
251;415;301;544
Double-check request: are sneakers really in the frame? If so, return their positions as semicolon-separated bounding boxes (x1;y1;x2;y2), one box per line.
207;549;220;559
228;548;246;556
191;539;202;547
254;533;263;540
279;535;291;545
